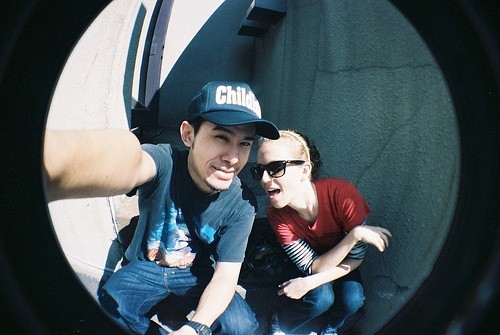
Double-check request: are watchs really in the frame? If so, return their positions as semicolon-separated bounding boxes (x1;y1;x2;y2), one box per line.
185;321;213;335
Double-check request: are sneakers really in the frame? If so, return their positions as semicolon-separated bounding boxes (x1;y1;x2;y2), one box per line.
270;312;286;335
321;319;338;335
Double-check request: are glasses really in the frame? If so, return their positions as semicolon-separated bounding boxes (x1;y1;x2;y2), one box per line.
251;160;305;180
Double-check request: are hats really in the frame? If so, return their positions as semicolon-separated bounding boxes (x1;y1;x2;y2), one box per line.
187;80;280;140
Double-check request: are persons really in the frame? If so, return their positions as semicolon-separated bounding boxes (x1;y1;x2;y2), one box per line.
44;81;280;335
250;130;393;335
